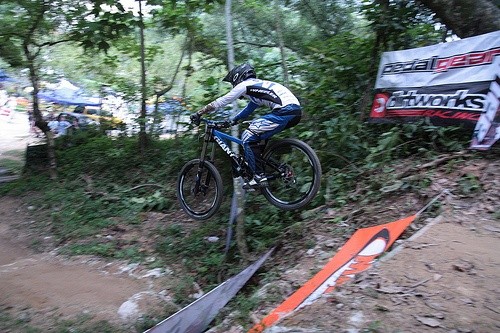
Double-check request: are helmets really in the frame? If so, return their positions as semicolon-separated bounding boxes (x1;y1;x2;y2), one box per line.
222;63;256;88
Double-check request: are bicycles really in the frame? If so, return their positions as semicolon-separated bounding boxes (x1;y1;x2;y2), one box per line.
177;116;321;221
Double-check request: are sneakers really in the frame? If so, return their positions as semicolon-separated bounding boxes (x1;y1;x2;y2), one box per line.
242;173;269;188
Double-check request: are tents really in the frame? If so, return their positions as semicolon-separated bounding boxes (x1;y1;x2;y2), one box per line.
0;71;188;137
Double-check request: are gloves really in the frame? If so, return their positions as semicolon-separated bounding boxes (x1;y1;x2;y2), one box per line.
190;112;200;123
223;118;233;128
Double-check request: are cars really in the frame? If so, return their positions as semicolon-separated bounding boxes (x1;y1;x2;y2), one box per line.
72;105;125;130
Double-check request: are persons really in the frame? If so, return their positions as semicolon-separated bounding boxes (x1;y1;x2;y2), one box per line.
28;105;96;141
189;64;301;183
